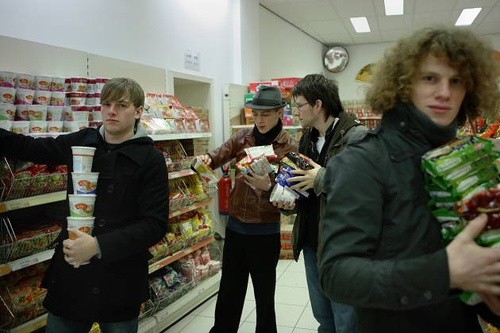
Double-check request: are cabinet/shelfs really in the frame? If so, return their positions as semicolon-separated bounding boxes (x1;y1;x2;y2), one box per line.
0;132;160;333
148;132;222;332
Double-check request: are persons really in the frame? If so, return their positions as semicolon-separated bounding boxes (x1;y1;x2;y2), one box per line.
317;28;499;333
1;77;171;333
273;72;374;333
198;84;299;333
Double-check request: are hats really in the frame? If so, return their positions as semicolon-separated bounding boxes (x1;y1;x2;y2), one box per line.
243;86;287;110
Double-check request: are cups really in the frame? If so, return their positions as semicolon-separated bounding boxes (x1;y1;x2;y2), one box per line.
270;183;295;206
71;146;96;172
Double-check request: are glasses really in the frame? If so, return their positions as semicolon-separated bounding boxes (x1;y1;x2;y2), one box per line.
296;101;309;109
